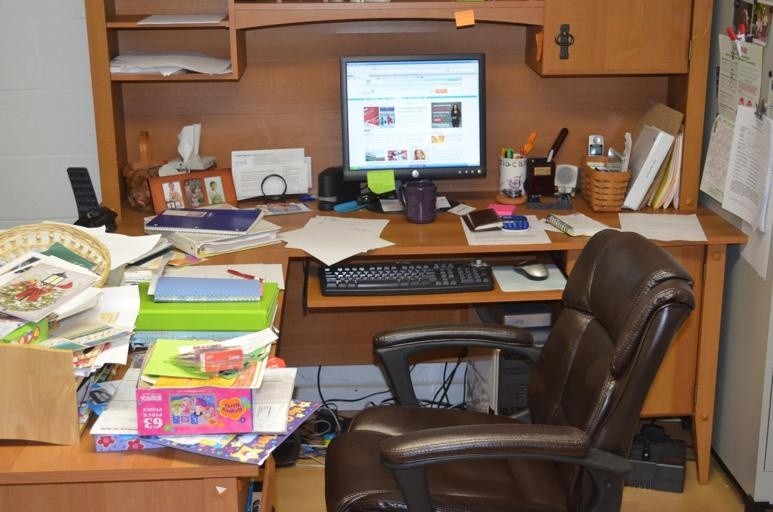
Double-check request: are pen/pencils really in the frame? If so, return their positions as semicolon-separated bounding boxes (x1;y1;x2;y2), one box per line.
501;132;536;159
498;215;527;221
228;268;263;282
546;128;569;163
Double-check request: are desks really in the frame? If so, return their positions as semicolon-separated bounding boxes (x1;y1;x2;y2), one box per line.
0;191;749;512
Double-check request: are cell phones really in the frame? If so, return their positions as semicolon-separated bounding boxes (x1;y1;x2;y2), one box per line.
587;134;605;157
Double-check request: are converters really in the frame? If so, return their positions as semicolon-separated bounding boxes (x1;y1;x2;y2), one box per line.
317;408;338;433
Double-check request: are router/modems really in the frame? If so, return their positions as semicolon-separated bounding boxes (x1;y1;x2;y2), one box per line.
492;301;553;329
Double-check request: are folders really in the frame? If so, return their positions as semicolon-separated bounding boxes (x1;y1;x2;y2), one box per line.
144;201;282;259
623;126;675;211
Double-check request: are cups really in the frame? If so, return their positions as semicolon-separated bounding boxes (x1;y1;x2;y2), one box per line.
498;152;527;200
397;180;437;224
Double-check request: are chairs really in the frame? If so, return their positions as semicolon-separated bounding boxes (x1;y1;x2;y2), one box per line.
323;227;698;512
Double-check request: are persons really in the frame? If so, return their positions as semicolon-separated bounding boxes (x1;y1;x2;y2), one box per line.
194;184;203;201
736;8;750;35
188;192;202;208
449;103;460;128
184;181;193;196
414;150;425;160
754;9;764;36
167;183;183;209
207;181;223;204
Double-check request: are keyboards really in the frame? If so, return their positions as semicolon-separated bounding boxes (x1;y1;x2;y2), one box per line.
319;260;494;296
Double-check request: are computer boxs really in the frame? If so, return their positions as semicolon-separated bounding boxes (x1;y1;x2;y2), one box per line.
463;306;554;424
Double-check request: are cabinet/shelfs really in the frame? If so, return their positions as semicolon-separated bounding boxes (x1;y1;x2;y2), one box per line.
86;1;713;223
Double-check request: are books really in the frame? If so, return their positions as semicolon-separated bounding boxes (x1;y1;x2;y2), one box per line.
545;211;609;237
0;251;135;378
462;207;503;234
621;100;684;212
144;203;282;258
134;276;280;331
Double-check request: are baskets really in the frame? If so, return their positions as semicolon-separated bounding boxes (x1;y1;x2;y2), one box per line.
0;223;112;289
579;154;632;213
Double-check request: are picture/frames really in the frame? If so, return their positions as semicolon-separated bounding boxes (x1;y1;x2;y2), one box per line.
146;168;239;216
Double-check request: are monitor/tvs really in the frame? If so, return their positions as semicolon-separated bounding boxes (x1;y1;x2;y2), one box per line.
339;53;487;214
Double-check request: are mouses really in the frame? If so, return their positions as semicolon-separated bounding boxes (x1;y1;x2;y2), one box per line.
514;258;549;280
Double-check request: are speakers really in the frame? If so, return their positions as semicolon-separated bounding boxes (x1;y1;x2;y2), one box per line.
553;163;581;198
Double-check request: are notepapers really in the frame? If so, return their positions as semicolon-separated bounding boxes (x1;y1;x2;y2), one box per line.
488;203;516;216
